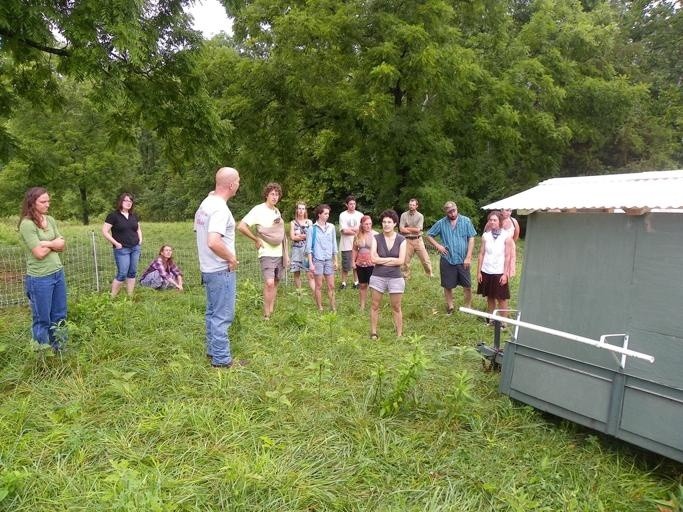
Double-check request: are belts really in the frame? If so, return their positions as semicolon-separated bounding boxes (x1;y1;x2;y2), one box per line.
404;235;421;240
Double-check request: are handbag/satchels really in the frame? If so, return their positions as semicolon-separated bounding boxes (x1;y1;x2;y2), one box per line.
304;225;316;269
255;218;285;247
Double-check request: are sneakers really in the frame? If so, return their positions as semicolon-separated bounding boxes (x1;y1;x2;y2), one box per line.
205;350;215;360
351;283;359;289
339;283;346;290
214;359;250;368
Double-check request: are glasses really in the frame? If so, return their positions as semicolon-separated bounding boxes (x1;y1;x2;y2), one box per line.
122;199;131;204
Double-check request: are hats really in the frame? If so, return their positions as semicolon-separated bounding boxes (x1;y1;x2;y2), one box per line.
443;201;460;211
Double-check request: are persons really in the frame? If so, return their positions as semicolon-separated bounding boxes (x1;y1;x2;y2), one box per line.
483;208;519;278
102;192;143;298
399;198;435;280
16;186;67;350
139;244;182;291
425;201;476;316
237;183;290;320
338;197;364;290
290;201;315;290
306;204;339;312
194;166;251;367
351;214;380;311
369;210;406;339
476;212;512;330
